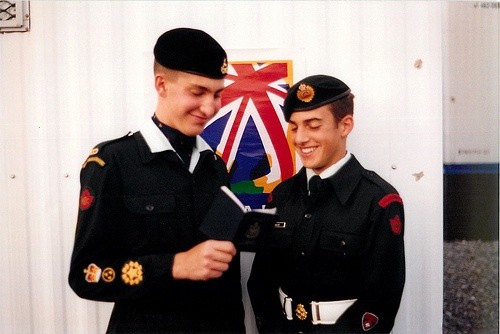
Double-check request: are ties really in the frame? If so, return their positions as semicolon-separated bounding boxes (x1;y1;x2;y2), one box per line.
308;174;322;200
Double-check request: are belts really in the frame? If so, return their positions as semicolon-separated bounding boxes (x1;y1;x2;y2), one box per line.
278;287;357;326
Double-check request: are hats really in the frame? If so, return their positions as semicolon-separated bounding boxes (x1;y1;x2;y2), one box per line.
154;28;228;79
278;74;350;122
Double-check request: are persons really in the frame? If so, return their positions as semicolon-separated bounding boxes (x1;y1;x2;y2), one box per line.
67;24;270;334
244;72;408;334
